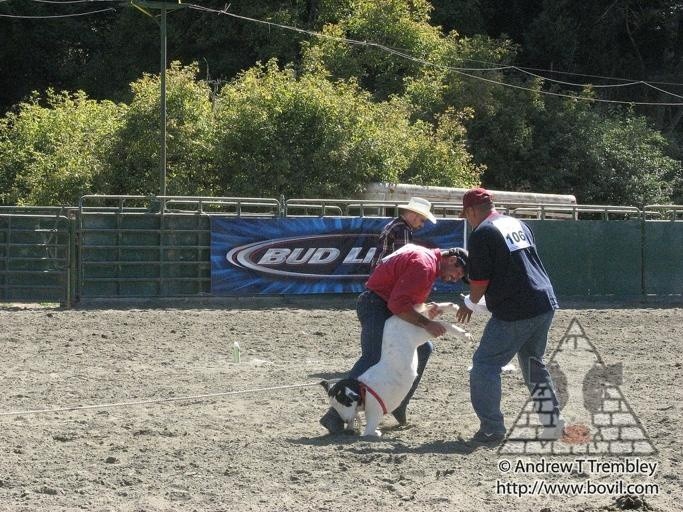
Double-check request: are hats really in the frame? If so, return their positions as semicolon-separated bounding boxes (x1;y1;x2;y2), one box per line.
397;197;437;225
458;188;493;218
449;248;470;284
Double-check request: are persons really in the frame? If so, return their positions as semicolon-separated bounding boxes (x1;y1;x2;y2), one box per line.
456;188;560;445
370;197;437;273
318;243;469;434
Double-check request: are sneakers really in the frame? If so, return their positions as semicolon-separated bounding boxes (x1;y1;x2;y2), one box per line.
392;404;406;426
319;412;354;435
473;430;505;443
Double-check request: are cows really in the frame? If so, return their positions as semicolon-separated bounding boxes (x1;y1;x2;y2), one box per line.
318;301;477;438
